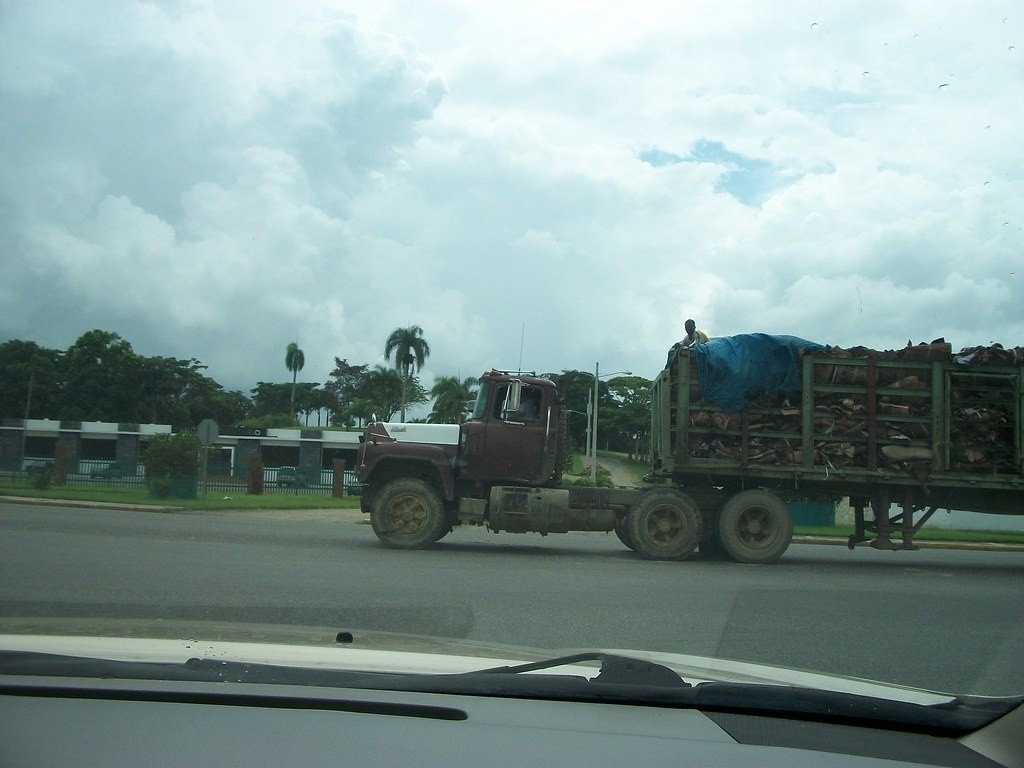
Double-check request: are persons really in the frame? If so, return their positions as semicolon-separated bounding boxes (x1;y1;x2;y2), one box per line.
682;320;709;348
511;389;536;420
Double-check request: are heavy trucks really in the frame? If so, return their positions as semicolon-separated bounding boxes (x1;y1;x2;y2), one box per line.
354;342;1024;564
